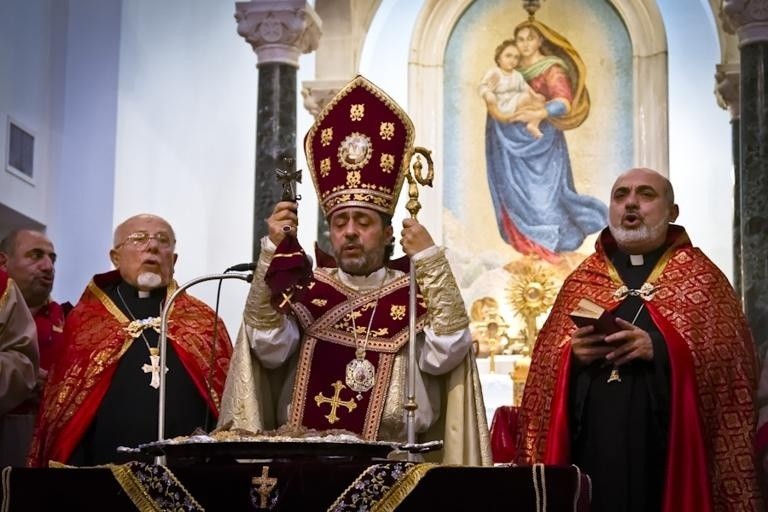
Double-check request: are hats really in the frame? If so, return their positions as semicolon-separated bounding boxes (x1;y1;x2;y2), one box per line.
303;74;416;220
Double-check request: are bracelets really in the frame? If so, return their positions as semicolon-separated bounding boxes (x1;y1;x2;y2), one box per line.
543;100;568;119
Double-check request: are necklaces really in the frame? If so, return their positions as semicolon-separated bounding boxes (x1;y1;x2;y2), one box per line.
335;266;388;393
606;258;644;383
117;285;169;390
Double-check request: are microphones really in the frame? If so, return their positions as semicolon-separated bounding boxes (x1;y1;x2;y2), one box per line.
231;254;314;272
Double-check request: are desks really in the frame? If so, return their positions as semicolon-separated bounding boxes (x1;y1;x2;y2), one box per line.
3;446;601;512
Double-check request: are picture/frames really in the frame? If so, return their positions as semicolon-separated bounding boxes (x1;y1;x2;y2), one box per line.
405;1;666;375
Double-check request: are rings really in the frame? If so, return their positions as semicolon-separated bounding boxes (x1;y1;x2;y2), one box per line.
282;225;291;235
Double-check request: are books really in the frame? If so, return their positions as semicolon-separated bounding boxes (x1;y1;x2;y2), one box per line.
568;298;628;348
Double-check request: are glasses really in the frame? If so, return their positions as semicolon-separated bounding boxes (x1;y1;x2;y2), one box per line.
115;232;171;250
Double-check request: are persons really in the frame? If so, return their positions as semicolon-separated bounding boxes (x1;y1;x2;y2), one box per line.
25;214;233;468
0;228;74;467
478;39;545;140
515;167;768;512
216;75;493;468
484;21;610;266
0;266;41;415
